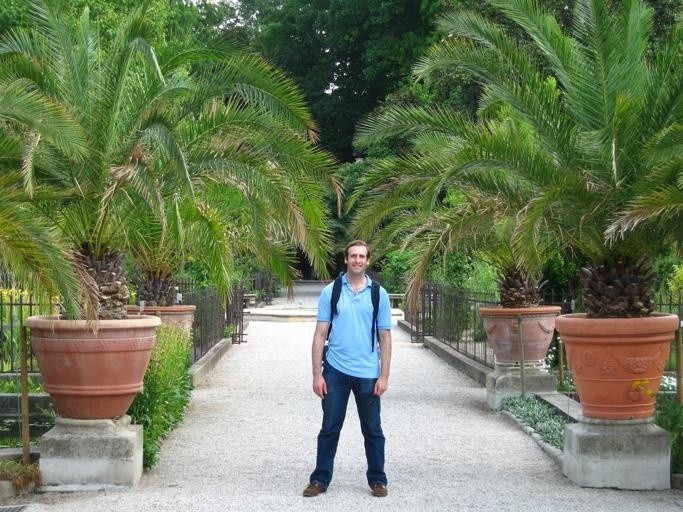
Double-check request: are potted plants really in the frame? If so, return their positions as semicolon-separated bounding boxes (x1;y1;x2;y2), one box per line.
353;80;589;367
112;68;253;349
1;2;341;421
354;1;683;420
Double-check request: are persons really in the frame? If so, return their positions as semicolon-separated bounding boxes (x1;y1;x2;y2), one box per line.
303;241;392;498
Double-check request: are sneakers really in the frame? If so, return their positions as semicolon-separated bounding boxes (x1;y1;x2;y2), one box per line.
372;481;387;497
303;482;327;496
